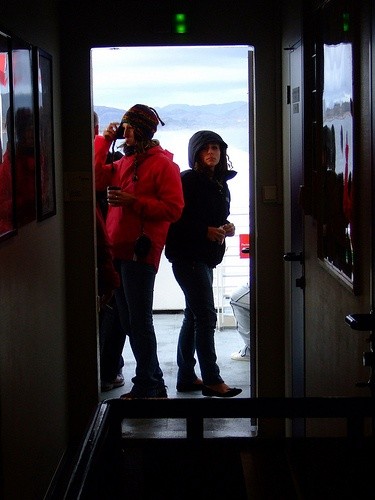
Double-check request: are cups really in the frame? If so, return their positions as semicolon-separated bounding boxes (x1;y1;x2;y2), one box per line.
107;186;121;205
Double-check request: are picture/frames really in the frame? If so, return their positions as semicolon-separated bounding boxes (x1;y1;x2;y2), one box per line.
312;0;363;298
0;30;58;243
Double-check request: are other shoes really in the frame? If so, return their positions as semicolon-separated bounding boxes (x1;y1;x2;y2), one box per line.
120;385;167;400
177;382;204;392
99;375;125;392
202;386;242;397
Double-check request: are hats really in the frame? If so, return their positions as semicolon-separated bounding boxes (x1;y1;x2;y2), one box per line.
109;104;165;183
16;107;35;155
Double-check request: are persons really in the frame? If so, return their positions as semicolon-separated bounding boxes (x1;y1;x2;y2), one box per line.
94;105;183;398
0;106;55;235
165;130;242;396
